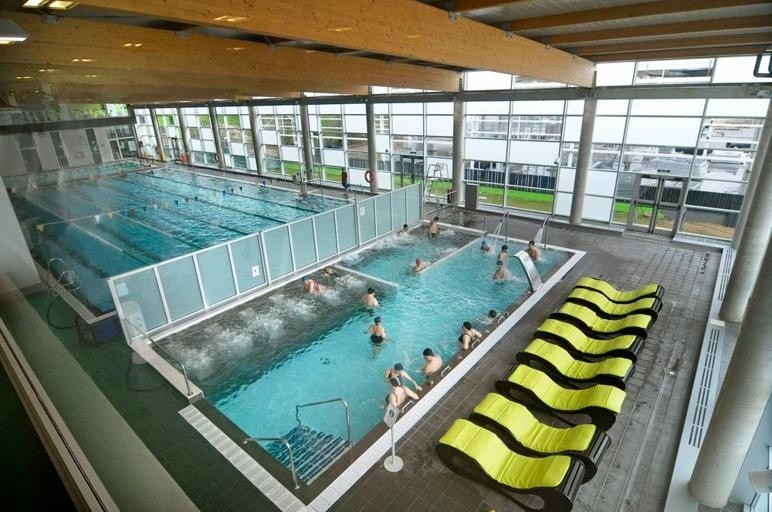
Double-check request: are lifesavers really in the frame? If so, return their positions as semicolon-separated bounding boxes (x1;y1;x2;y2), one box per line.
155;147;161;155
214;153;220;162
75;150;85;159
365;170;374;183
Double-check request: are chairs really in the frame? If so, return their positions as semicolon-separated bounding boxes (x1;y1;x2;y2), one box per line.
434;419;587;512
573;277;665;304
494;362;628;431
549;301;658;340
516;335;637;390
565;286;663;323
469;390;612;484
533;317;646;362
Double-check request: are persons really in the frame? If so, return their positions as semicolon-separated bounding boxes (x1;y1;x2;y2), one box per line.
496;244;508;265
363;316;386;347
488;309;496;318
527;240;541;263
361;286;378;306
429;216;440;237
324;267;339;280
413;259;425;271
492;261;506;280
380;363;423;410
398;224;410;238
480;239;492;253
458;321;482;350
301;276;321;294
420;348;443;376
341;167;348;195
